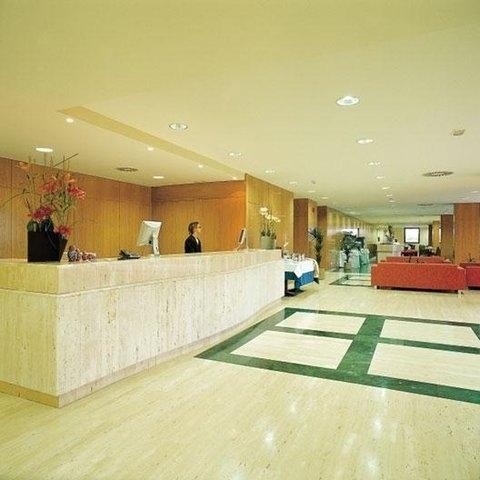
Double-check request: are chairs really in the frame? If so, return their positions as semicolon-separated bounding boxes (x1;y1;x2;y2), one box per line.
459;262;480;287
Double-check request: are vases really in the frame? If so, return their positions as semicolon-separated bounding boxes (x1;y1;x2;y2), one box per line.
28;231;68;262
261;237;275;249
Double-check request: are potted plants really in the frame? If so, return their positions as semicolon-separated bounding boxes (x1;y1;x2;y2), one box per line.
338;231;361;270
307;227;326;279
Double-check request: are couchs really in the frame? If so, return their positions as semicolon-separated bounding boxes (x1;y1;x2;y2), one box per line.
371;256;466;294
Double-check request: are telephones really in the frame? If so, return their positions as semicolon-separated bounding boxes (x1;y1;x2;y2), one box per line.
118;249;140;260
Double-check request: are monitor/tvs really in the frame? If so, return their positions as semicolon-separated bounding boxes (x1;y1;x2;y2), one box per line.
136;221;162;256
238;228;248;250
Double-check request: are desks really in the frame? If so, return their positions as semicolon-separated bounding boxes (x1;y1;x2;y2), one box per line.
285;257;319;296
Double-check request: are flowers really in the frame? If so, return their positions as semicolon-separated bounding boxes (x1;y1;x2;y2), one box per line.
2;152;85;238
259;207;281;239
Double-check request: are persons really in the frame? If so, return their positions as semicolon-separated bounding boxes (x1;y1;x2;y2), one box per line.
184;222;203;252
393;238;417;258
